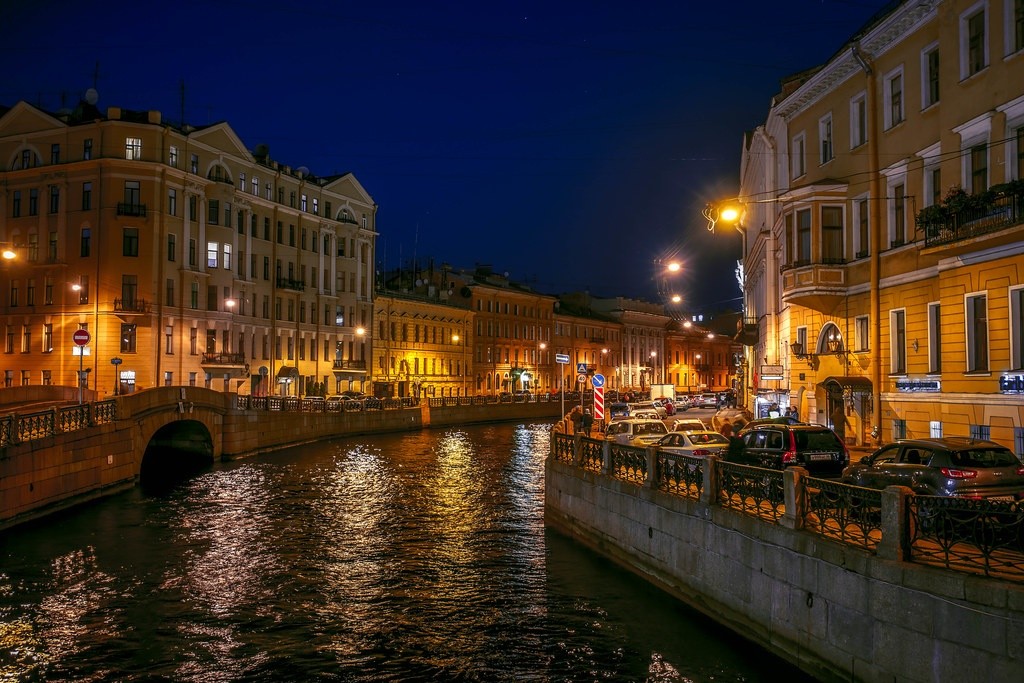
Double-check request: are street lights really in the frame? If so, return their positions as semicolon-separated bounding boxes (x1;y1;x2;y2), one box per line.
535;340;546;401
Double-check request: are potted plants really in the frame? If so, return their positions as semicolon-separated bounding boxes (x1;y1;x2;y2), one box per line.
914;205;945;237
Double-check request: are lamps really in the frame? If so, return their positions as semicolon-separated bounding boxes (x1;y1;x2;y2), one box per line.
827;338;851;354
790;340;812;361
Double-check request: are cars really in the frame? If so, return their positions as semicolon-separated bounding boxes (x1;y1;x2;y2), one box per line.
719;391;731;405
605;395;675;435
498;390;531;401
285;395;381;409
675;395;701;411
648;429;730;471
553;389;639;398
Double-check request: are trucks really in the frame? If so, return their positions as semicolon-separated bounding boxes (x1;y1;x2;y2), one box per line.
650;383;677;404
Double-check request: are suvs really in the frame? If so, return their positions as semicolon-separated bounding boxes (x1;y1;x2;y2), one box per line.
699;393;720;409
723;421;849;488
672;418;710;442
615;417;671;458
841;435;1024;534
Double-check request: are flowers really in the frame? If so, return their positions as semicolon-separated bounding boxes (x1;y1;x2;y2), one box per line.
943;184;970;218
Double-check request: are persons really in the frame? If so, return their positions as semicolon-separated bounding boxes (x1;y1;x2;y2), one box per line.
715;390;749;463
570;406;594;439
767;403;799;424
829;408;851;443
407;393;411;406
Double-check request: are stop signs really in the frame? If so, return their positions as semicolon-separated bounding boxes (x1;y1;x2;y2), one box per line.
73;329;90;346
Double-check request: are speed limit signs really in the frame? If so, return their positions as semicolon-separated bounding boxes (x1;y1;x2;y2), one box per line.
577;375;587;383
